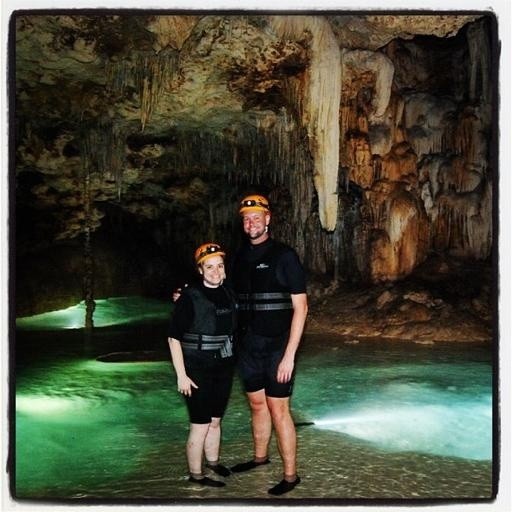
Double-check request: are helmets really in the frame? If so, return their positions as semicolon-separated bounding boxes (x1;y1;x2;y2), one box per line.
239;195;271;216
194;243;226;267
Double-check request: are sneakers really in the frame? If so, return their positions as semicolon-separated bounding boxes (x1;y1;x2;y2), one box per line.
205;463;230;477
268;475;300;496
231;458;270;473
189;475;226;487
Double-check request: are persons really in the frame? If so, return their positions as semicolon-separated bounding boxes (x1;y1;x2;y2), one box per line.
171;193;309;496
167;241;237;487
